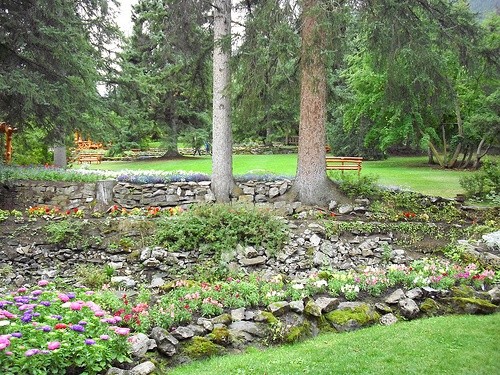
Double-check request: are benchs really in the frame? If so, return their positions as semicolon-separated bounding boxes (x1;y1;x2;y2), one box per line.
326;156;363;179
76;152;105;165
